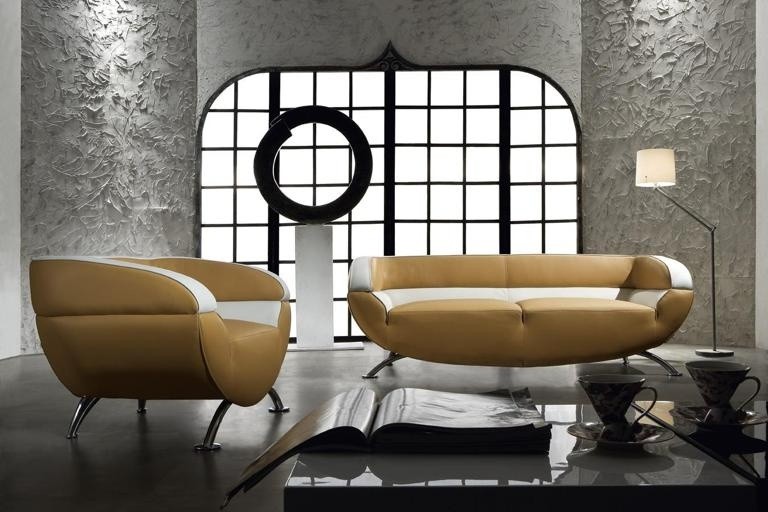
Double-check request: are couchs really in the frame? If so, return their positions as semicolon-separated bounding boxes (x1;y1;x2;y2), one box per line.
346;250;696;380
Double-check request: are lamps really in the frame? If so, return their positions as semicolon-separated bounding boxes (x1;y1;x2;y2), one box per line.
632;146;737;359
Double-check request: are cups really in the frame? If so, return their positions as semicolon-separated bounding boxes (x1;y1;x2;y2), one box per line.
684;357;761;417
577;372;660;435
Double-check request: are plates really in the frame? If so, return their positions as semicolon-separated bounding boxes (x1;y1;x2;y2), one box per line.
669;404;766;432
565;421;678;450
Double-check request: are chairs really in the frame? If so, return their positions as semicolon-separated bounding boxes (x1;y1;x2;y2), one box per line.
28;252;290;452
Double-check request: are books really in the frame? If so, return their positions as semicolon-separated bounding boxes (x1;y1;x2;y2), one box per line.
219;387;552;508
